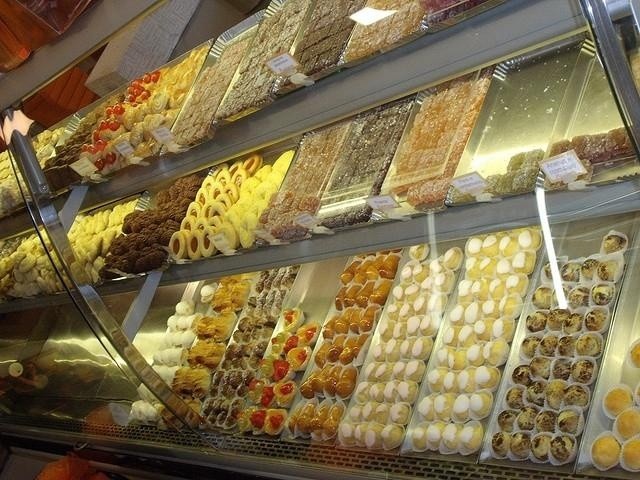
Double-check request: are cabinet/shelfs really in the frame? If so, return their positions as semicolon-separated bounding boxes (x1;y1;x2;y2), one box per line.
2;0;640;479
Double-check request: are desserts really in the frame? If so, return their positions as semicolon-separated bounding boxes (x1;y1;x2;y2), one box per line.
1;0;640;469
